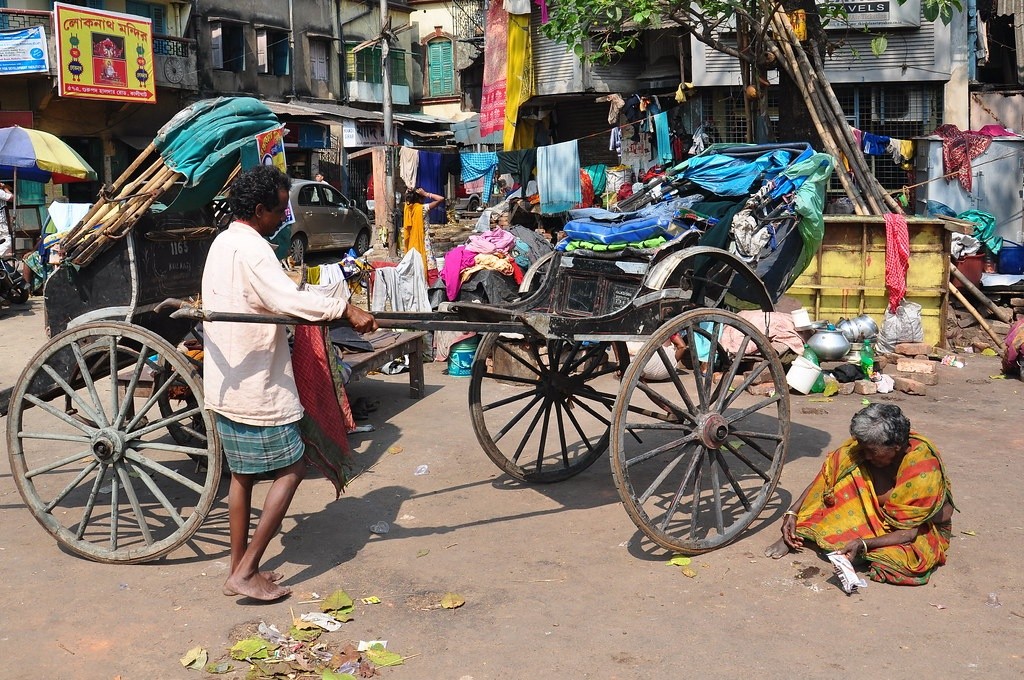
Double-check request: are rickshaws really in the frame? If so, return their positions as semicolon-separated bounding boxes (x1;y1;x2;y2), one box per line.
152;140;835;556
0;99;297;566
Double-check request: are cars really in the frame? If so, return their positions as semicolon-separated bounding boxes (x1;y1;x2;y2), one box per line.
213;178;372;267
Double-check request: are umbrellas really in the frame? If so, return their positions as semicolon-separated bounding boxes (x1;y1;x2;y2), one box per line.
0;124;98;268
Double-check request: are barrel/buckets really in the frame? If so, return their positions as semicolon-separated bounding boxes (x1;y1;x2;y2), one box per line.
997;239;1024;275
948;251;985;307
448;337;476;377
785;355;822;394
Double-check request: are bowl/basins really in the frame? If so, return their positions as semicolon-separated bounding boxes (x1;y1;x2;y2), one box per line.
836;314;879;342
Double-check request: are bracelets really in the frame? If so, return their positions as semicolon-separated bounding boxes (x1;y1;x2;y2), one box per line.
782;511;798;520
427;192;432;199
858;536;868;558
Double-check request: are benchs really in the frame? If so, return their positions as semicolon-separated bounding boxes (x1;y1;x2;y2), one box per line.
527;200;737;316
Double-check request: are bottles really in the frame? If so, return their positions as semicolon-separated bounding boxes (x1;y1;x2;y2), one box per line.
803;344;824;392
860;340;874;381
807;329;851;361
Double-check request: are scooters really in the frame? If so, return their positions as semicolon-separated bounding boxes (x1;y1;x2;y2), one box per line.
0;238;32;319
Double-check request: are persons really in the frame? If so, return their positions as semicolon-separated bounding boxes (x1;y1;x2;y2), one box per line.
310;171;333;203
764;403;960;586
400;186;444;270
202;166;378;602
21;196;69;298
0;182;14;239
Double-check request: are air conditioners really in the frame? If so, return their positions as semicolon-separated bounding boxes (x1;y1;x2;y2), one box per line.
870;82;930;121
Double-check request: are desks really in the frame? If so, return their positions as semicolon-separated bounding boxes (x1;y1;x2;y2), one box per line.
108;332;428;437
